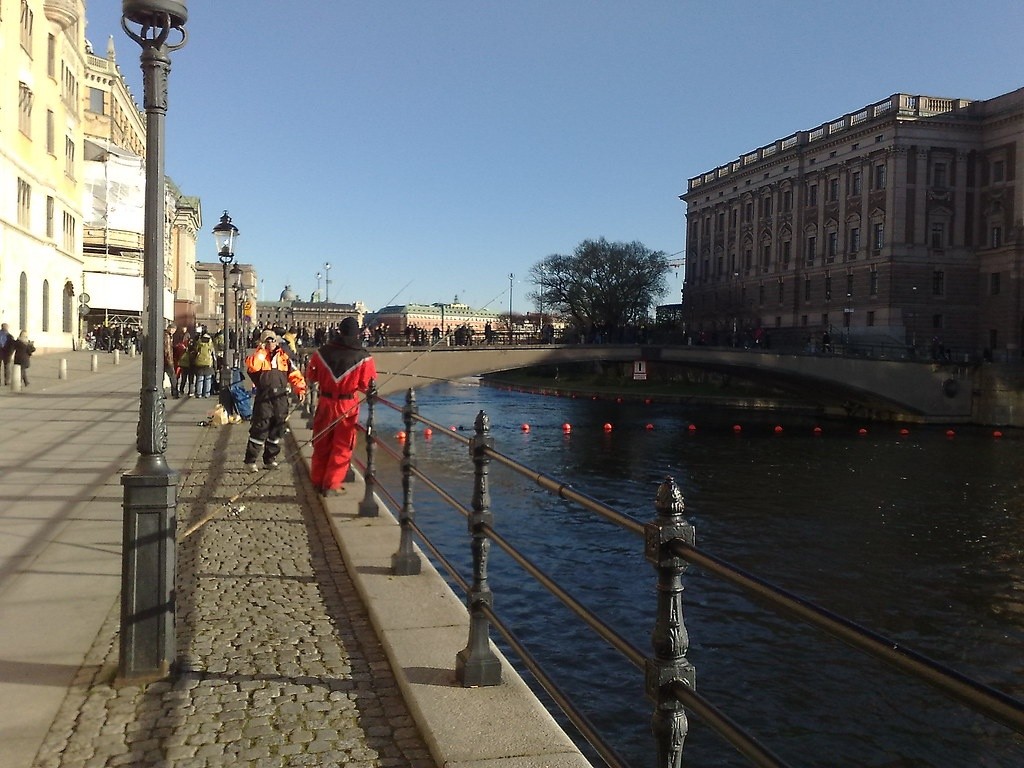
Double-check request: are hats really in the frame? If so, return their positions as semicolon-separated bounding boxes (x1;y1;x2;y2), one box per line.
338;317;360;335
260;330;277;342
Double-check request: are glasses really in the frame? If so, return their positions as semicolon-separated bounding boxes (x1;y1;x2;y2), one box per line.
266;340;277;343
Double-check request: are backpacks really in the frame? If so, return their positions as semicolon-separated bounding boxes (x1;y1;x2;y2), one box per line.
209;404;228;427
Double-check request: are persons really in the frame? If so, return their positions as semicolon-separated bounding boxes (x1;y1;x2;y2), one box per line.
759;328;767;348
163;323;225;399
480;321;493;346
823;331;831;353
403;324;428;346
94;323;143;354
694;329;706;344
244;329;307;474
230;322;389;394
541;324;554;344
446;323;475;346
0;323;33;386
307;317;377;496
431;324;442;340
591;323;602;344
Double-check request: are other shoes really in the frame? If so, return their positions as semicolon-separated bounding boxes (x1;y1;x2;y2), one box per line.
261;462;280;470
244;463;257;473
324;488;348;497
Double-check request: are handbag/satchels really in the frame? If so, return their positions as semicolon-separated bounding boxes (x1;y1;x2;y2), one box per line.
27;340;32;356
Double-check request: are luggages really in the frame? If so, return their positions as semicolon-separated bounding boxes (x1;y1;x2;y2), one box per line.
225;384;253;420
